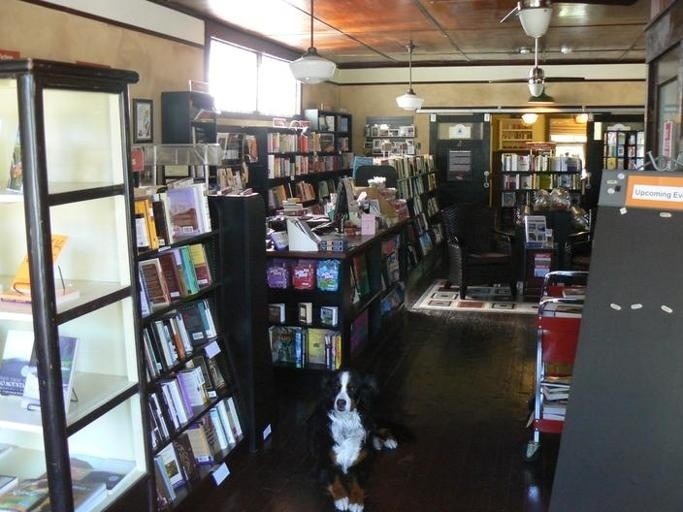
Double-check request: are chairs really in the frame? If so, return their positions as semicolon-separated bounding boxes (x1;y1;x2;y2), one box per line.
441;202;526;300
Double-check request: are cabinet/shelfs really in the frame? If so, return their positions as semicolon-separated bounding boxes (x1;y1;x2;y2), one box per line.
0;58;269;512
249;107;444;391
492;114;648;300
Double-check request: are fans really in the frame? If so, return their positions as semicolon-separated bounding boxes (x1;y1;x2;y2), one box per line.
498;0;637;39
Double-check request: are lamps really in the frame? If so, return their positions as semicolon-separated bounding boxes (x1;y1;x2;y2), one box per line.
509;38;554;104
287;0;337;86
394;39;425;111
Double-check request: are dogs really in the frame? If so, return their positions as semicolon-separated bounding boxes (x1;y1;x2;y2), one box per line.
304;367;399;512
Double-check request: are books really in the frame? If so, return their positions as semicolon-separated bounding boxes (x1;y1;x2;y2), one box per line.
1;283;81;309
500;151;586;422
603;131;644;170
19;332;80;421
6;124;24;195
0;442;137;511
11;230;71;293
1;327;35;398
